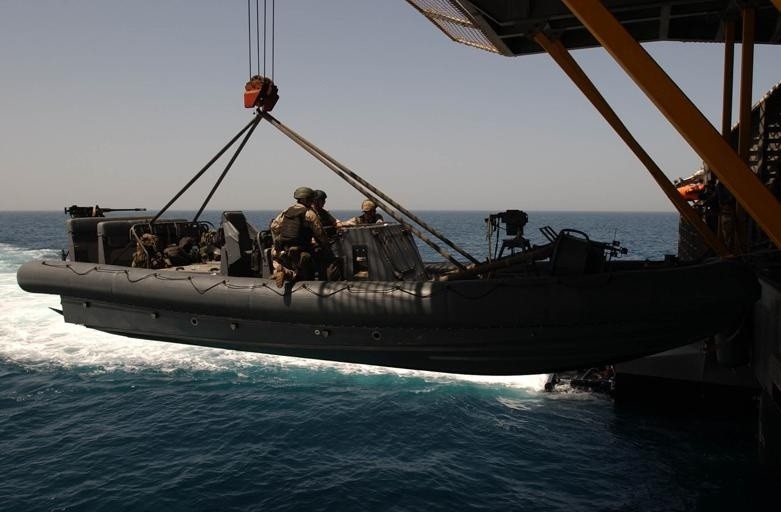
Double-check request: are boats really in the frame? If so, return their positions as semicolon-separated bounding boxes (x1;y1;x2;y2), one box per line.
15;195;746;376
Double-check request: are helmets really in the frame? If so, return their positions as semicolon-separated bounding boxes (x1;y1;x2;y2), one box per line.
294;186;327;201
360;198;377;212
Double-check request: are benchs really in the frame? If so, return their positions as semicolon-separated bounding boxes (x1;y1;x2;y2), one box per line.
128;218;217;268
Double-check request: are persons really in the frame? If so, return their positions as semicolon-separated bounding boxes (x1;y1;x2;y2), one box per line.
129;182;384;293
